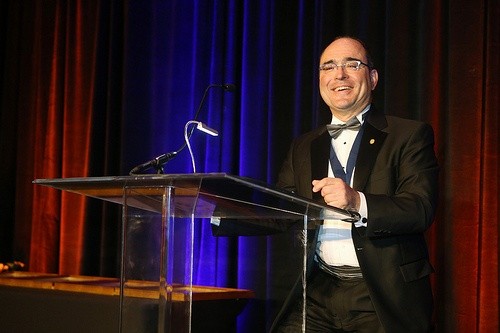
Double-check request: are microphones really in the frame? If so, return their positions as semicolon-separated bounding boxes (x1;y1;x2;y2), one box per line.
131;80;236;176
185;119;219;175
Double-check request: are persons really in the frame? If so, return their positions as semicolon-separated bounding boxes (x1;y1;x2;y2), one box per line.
208;38;437;333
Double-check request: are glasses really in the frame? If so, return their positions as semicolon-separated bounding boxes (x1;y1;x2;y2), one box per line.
318;60;370;71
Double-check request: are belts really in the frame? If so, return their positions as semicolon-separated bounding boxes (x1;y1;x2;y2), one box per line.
315;251;363;280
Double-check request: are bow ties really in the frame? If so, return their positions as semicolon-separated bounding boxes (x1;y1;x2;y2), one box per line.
326;116;362;139
